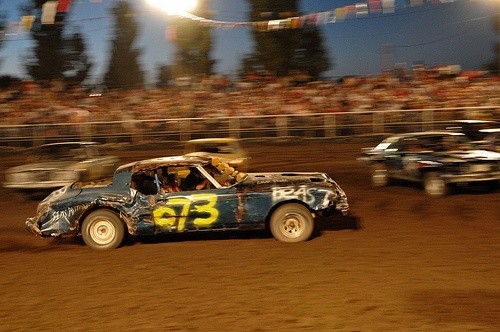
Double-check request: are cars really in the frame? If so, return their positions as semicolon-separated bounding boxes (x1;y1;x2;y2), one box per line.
26;156;349;250
356;129;500;198
2;142;124;196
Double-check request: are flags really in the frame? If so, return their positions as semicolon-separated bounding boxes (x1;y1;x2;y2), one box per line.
0;0;457;39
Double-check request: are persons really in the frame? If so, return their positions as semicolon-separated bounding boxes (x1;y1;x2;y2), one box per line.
184;167;213;190
167;174;182;192
0;63;500;130
